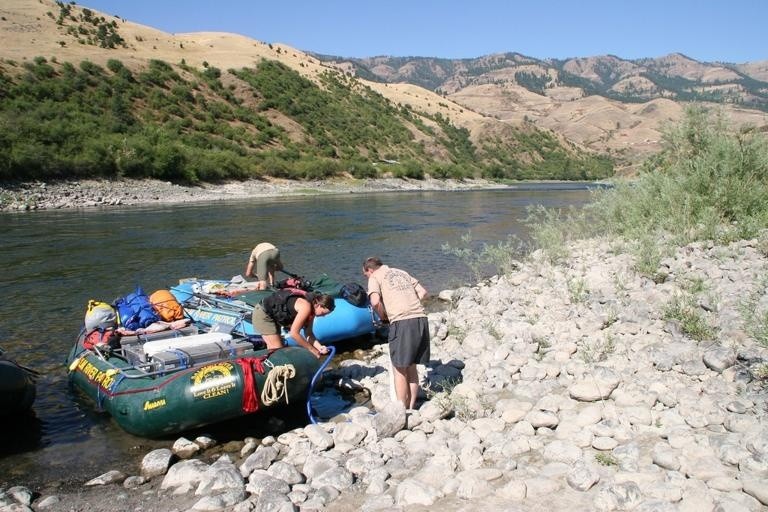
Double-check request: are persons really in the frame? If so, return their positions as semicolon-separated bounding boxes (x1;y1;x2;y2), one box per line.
245;243;283;287
252;289;335;359
362;256;430;410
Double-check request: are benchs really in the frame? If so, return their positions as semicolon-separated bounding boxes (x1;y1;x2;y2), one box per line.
153;339;255;377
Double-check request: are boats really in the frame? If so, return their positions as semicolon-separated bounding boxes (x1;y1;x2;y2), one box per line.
160;277;389;357
0;345;38;428
63;289;325;440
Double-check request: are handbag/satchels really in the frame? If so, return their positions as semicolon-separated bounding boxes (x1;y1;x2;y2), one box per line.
338;281;367;307
